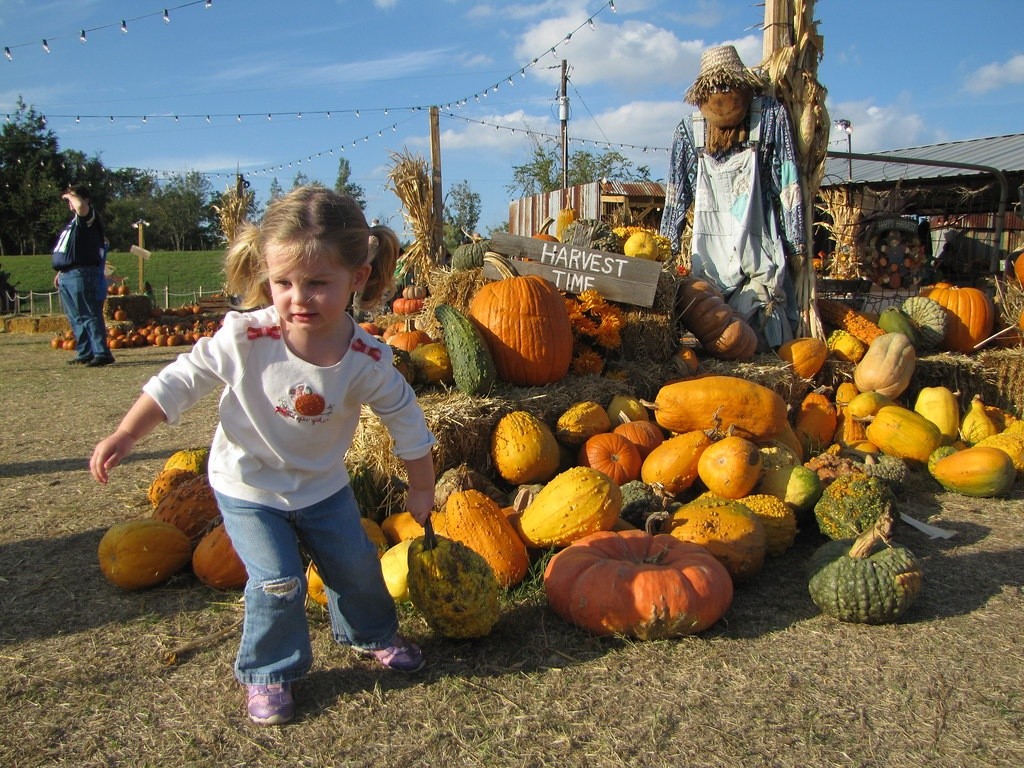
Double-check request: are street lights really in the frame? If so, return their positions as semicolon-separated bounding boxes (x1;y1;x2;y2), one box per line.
834;119;853;205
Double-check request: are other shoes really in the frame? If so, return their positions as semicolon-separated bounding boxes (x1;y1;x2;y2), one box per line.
87;354;115;368
67;355;92;365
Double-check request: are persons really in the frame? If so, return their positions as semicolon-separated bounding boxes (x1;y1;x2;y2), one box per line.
87;182;440;727
52;183;117;367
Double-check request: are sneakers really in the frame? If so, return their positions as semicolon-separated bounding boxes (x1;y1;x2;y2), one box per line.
246;678;295;726
350;636;426;673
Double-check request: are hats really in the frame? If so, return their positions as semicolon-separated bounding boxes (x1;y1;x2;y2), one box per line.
684;44;773;104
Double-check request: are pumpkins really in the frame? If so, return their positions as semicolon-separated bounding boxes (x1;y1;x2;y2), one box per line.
50;194;1024;638
